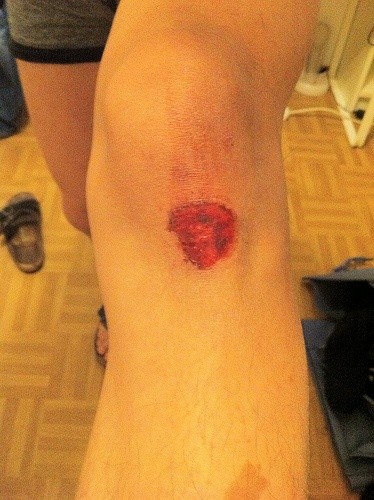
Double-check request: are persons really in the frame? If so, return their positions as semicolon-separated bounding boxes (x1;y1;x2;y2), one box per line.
4;0;320;500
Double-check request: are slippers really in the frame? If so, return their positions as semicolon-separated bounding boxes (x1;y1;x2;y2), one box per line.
1;192;44;272
94;304;110;370
